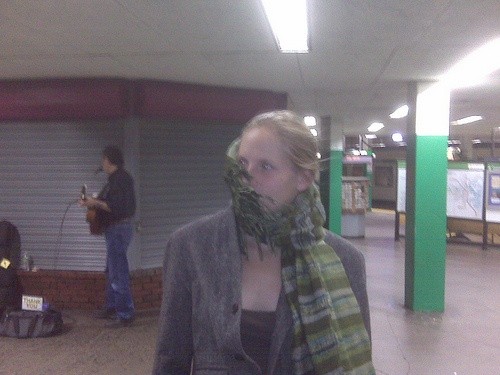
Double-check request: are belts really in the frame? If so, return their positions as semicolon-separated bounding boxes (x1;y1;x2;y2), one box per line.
104;217;130;225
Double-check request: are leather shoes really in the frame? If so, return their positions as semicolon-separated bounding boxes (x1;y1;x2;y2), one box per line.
95;310;117;320
106;317;132;328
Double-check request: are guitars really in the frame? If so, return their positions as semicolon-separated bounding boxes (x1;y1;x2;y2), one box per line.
81;183;105;234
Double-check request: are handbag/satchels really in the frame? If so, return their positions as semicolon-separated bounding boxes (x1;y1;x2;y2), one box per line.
1;306;62;338
